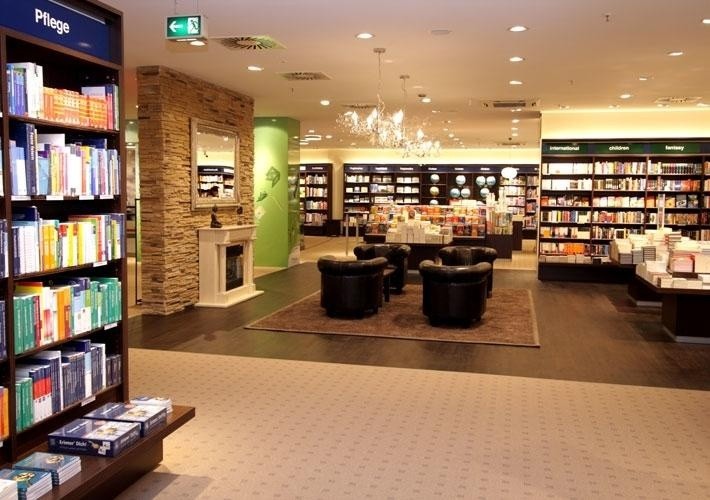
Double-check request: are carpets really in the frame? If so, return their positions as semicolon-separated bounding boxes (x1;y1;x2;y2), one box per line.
242;281;540;347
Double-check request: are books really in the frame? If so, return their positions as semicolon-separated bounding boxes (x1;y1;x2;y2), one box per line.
2;206;125;278
540;163;590;257
6;63;119;131
611;227;709;289
2;276;122;359
394;176;419;203
647;160;708;242
370;174;394;205
499;175;539;228
9;123;120;195
372;206;512;243
593;162;646;256
1;339;122;439
198;174;234;199
300;176;327;222
345;174;369;222
0;396;172;500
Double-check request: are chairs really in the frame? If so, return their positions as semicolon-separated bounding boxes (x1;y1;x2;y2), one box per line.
317;241;498;328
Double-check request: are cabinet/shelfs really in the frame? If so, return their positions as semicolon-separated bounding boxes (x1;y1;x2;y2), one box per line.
538;153;710;282
343;172;539;239
198;172;235;197
0;25;195;500
300;171;332;236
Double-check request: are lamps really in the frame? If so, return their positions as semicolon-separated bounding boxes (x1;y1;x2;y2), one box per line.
336;47;442;161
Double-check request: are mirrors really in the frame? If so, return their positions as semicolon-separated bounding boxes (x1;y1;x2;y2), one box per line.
189;116;241;212
